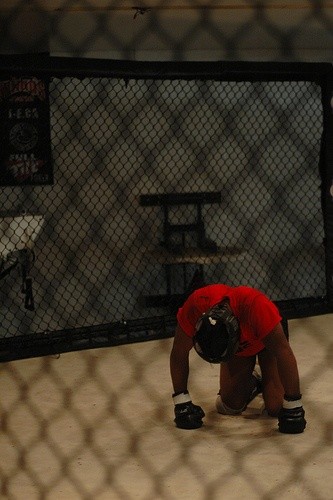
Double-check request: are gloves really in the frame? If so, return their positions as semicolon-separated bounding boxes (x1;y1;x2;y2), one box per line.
171;390;205;430
278;394;306;434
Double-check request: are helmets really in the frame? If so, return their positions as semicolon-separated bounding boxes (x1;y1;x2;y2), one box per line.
193;306;240;363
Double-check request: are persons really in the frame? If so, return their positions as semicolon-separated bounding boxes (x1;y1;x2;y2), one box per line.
169;284;307;435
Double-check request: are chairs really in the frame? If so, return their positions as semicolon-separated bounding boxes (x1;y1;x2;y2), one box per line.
134;189;248;311
